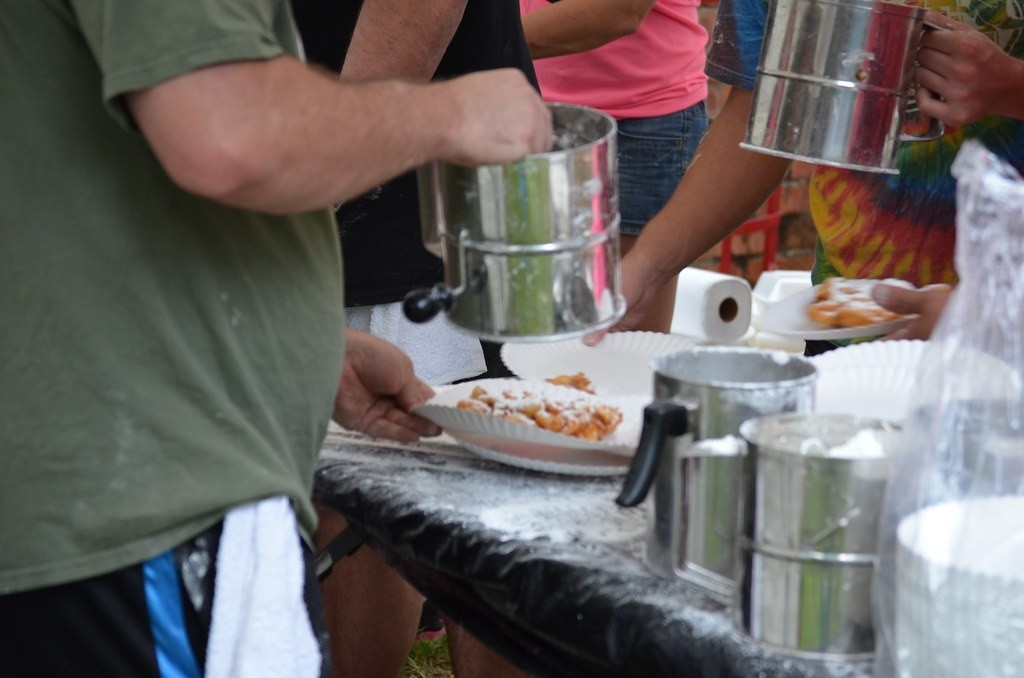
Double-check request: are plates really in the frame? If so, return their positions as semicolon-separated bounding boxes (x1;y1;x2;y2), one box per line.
814;340;1024;678
408;330;700;478
748;306;918;340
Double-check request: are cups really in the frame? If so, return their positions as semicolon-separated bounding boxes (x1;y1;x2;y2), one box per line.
739;2;945;177
668;411;904;661
617;347;819;580
406;104;626;339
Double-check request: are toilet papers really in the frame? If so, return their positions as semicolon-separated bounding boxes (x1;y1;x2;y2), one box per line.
669;266;753;341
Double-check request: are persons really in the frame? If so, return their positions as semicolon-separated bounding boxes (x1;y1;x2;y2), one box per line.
294;0;1024;678
0;0;550;678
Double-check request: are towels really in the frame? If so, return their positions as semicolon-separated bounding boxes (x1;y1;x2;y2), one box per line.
203;495;323;678
343;294;489;386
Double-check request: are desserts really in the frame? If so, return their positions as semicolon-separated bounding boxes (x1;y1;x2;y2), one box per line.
457;385;624;438
809;275;914;328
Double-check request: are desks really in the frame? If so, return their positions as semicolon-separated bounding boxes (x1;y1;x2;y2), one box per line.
300;299;874;675
